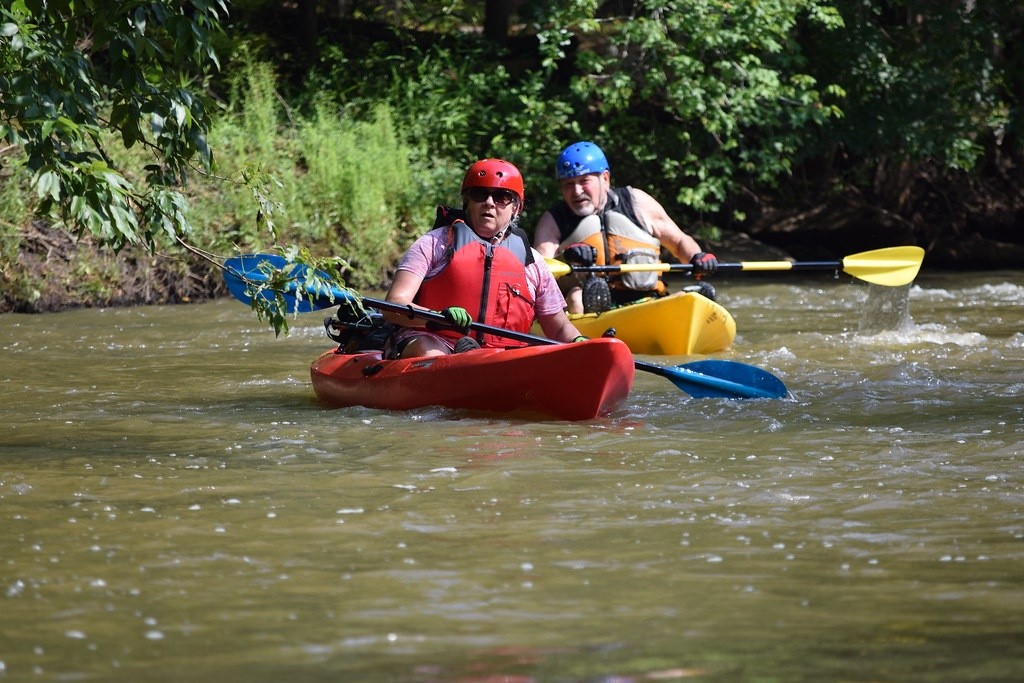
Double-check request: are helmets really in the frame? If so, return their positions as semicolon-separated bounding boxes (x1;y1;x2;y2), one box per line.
556;142;609;180
462;159;525;214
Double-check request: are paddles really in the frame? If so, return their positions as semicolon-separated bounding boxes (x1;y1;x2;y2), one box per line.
219;251;792;404
535;242;930;289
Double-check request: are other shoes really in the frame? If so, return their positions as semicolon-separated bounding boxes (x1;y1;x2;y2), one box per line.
454;337;482;353
582;277;610;312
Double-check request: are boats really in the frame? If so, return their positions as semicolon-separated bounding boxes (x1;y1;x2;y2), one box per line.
530;289;738;356
311;298;635;421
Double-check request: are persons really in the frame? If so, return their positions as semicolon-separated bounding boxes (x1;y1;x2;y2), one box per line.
534;142;717;313
382;159;588;360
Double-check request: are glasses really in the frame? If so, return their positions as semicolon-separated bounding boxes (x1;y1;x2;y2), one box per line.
469;187;517;206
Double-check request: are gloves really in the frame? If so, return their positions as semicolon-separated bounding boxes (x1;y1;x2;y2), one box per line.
429;306;474;336
687;252;717;273
562;242;597;266
571;335;589;342
